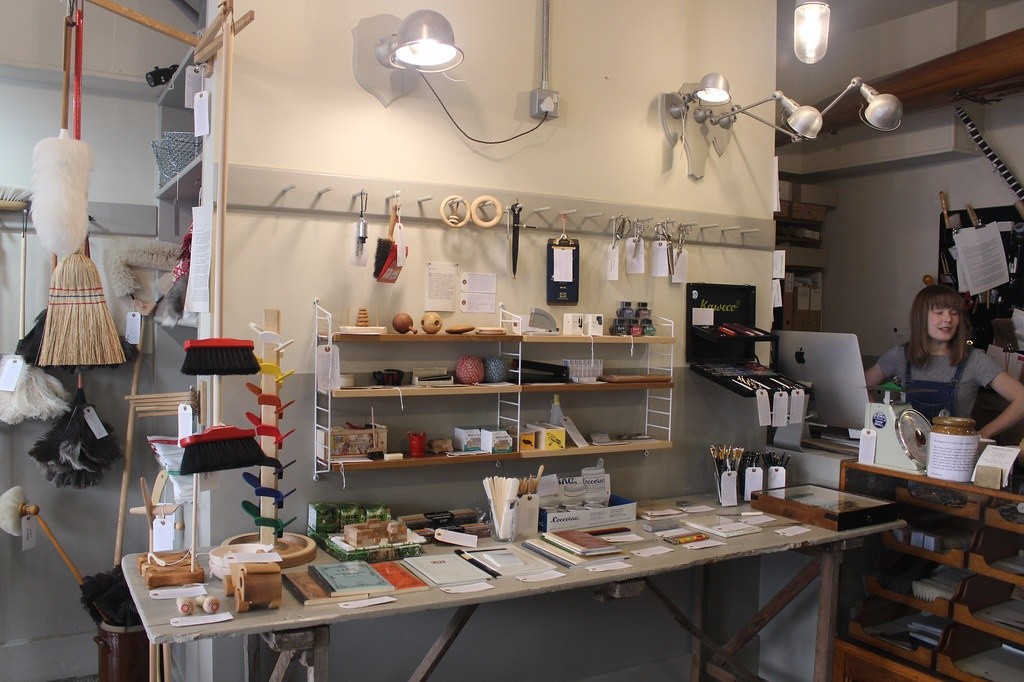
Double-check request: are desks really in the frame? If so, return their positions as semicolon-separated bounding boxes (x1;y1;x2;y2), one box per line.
122;490;907;682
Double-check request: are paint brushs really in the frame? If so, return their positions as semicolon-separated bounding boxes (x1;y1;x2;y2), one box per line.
710;445;744;490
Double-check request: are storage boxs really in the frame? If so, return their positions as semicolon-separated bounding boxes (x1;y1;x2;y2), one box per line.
538;475;638;534
563;314;603;337
315;424;388;461
773;180;837;333
454;423;566;454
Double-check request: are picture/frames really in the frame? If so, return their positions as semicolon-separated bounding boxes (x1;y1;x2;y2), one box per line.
752;484;899;532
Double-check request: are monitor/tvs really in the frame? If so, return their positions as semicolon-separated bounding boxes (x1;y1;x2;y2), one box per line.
771;329;871;452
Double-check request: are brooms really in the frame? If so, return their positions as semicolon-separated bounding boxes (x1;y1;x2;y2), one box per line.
179;22;262;376
0;184;33;211
15;9;140;371
179;200;268;476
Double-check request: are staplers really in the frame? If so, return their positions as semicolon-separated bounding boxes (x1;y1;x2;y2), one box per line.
522;307;561;336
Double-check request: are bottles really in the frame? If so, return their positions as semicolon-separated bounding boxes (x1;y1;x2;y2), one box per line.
924;417;979;482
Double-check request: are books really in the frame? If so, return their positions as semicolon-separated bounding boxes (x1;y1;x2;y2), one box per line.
543;529;620;556
682;515;762;537
908;615;952;646
401;554;492;587
284;559;429;604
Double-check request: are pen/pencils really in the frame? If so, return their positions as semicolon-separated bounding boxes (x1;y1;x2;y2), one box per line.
762;448;791;469
741;449;760;470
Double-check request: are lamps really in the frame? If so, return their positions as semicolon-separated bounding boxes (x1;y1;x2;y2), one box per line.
351;9;464;111
660;0;902;179
146;64;181;86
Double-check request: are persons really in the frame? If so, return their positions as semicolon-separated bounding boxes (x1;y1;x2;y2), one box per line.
864;286;1024;438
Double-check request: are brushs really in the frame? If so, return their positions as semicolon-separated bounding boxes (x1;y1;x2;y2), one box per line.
102;237;185;567
373;204;398;279
0;184;33;211
0;485;113;627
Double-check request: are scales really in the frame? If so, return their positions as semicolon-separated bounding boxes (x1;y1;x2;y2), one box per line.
864;381;931;476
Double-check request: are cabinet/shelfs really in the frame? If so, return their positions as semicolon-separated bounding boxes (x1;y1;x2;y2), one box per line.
832;453;1024;682
157;44;205;201
312;298;674;483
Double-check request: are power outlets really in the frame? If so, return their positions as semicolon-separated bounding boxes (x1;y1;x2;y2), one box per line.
530;89;560;121
